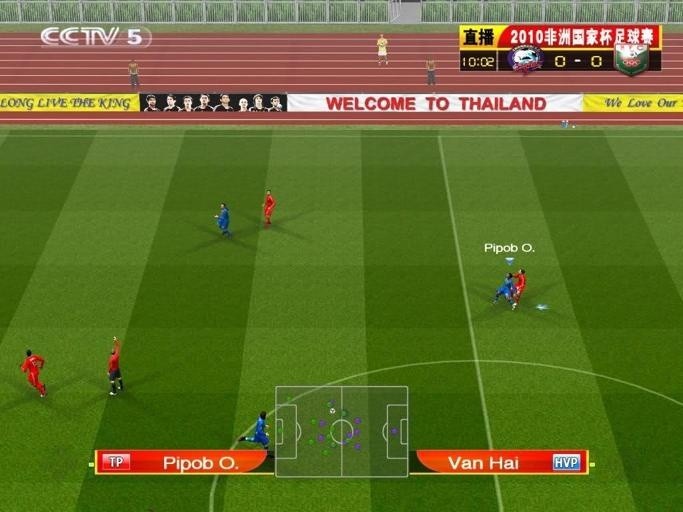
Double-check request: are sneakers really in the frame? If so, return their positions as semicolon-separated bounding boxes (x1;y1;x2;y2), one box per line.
239;435;246;441
512;302;517;311
266;455;274;458
109;387;121;396
40;384;45;397
